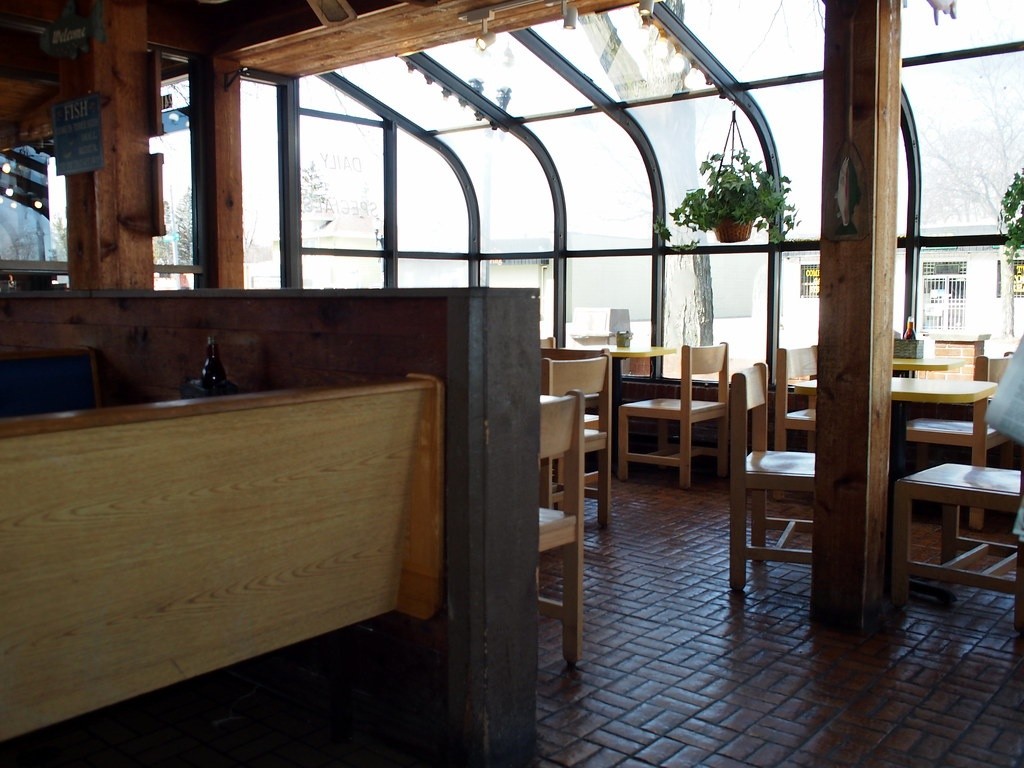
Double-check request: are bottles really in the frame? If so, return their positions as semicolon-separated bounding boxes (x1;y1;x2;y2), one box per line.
200;335;228;394
904;316;916;340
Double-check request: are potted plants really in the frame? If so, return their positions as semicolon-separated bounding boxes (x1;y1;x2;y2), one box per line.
652;146;803;257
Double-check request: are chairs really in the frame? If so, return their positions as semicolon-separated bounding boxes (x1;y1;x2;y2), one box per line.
540;351;614;527
889;428;1024;637
0;344;103;418
775;344;819;455
614;341;730;485
536;390;584;666
907;352;1016;532
727;360;822;594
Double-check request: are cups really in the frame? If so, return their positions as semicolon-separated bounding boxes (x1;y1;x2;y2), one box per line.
616;330;630;347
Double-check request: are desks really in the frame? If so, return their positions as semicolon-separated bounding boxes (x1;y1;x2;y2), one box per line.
579;344;676;464
892;356;970;379
793;375;999;526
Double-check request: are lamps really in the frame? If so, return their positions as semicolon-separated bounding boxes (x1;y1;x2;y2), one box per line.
457;0;654;52
